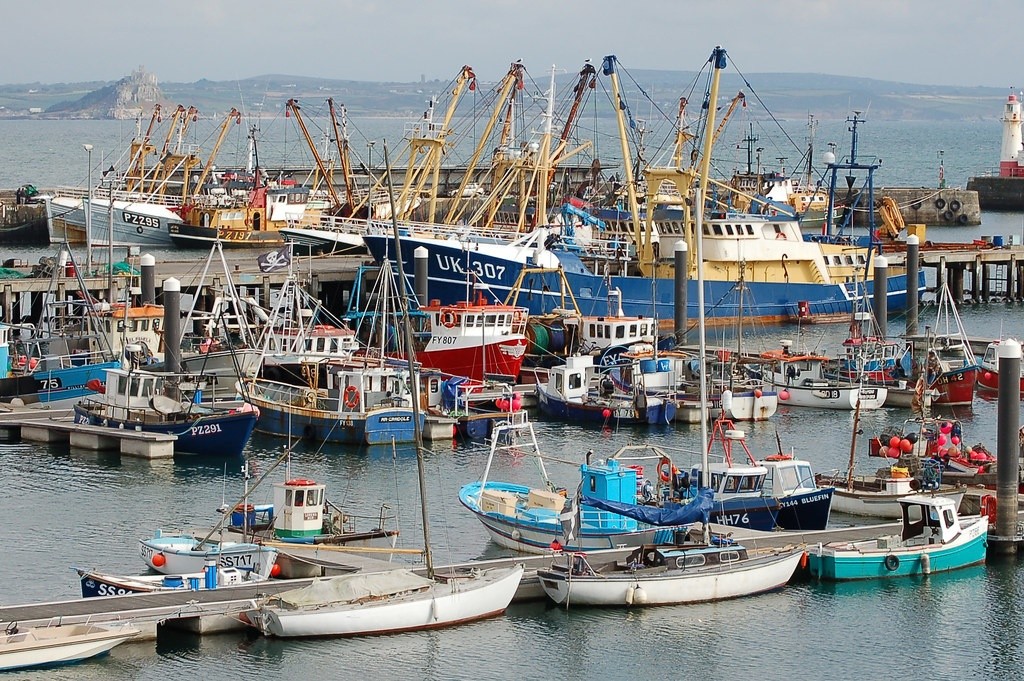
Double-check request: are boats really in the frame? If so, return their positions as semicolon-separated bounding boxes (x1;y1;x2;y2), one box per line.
0;619;141;672
809;490;989;582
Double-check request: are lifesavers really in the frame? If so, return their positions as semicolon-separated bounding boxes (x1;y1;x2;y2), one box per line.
440;308;459;330
654;455;678;484
512;308;524;323
773;230;789;242
313;323;337;331
343;383;362;410
764;452;794;462
979;494;1000;526
911;198;968;223
885;555;900;571
232;502;256;516
284;477;318;489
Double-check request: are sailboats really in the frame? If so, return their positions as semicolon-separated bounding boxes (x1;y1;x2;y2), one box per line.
536;186;809;608
0;46;1024;640
240;262;527;640
